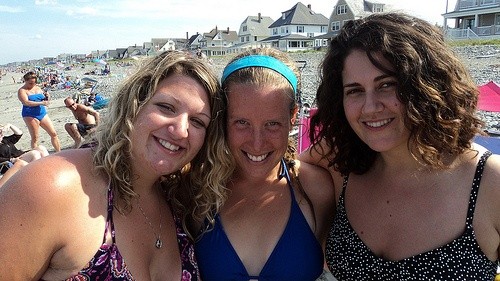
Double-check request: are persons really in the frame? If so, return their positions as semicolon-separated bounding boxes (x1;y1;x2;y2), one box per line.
164;46;336;281
195;47;206;61
32;68;96;104
64;97;100;149
0;48;236;281
101;63;110;74
18;71;61;153
171;12;500;281
0;123;49;186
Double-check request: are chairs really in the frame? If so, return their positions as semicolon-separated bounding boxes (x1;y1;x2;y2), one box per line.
298;107;324;154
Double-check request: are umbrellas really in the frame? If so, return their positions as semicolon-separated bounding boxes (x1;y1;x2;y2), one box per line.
92;59;106;65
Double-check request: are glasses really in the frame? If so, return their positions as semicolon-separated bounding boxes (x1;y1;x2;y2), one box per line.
68;103;74;108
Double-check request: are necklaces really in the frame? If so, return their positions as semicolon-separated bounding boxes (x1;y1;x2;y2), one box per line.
138;204;164;248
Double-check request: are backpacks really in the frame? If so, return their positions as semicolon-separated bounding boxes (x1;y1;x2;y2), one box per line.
93;99;109;110
